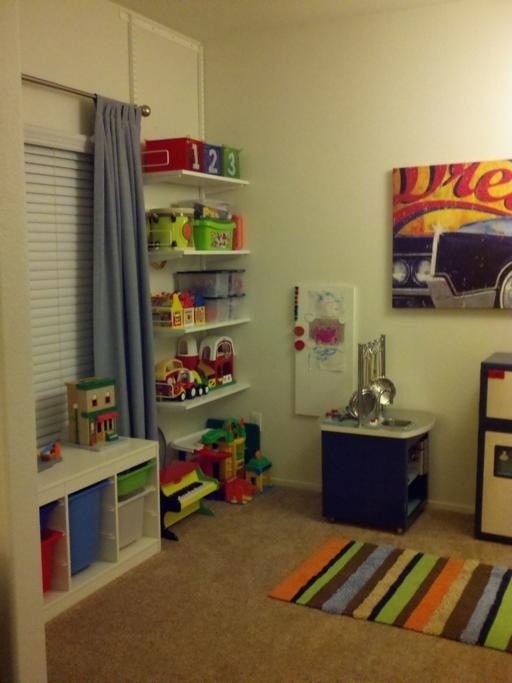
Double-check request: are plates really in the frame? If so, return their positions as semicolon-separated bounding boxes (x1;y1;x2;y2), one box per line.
139;170;255;409
473;351;512;545
320;407;435;532
34;434;161;624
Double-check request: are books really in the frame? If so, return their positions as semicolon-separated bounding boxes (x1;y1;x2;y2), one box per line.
143;138;240;179
169;428;215;462
38;457;156;594
172;269;244;323
192;219;236;251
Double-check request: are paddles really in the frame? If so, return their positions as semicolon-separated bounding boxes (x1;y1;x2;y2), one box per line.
267;534;512;653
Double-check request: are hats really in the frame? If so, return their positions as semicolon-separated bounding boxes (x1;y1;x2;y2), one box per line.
392;231;512;308
